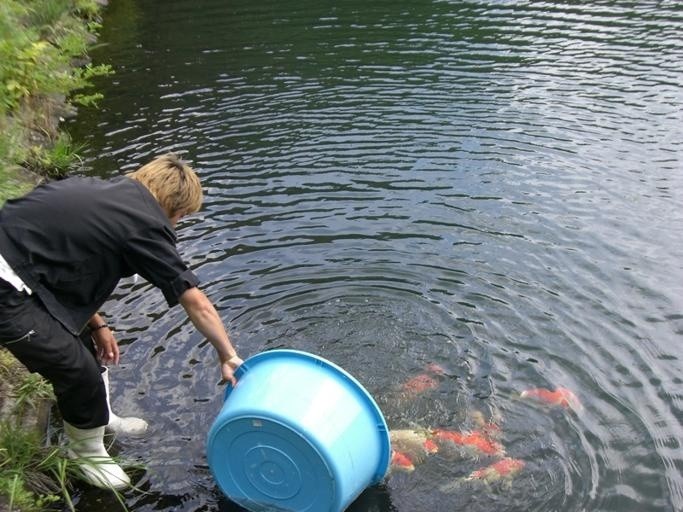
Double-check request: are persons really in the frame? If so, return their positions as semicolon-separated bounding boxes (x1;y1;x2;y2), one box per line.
0;155;245;494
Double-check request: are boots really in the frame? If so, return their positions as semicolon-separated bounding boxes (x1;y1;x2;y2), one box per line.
63;421;131;491
98;364;151;436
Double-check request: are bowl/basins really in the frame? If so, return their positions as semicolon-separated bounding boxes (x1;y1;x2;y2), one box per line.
206;350;392;512
